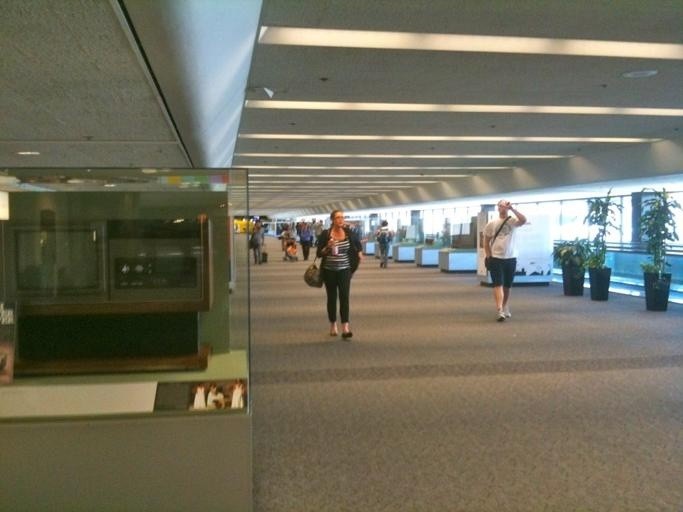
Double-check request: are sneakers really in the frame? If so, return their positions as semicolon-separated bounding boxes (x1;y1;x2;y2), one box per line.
340;332;353;340
495;306;511;322
328;329;338;337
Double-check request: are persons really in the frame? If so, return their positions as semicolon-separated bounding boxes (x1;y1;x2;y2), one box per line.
239;217;367;265
313;209;363;340
192;383;207;410
206;383;224;410
484;198;525;329
230;378;245;408
373;220;396;269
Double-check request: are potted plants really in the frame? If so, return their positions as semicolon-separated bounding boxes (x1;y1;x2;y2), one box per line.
636;188;683;311
583;187;624;301
551;237;586;296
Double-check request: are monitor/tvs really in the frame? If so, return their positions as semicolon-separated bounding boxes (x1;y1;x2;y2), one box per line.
0;221;107;297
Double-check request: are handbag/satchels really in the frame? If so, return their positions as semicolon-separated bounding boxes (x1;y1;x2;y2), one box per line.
303;263;323;288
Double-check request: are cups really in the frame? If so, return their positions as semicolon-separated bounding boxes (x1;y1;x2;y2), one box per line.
331;245;338;256
